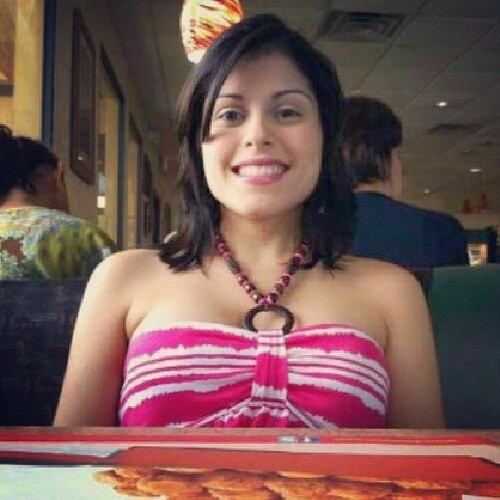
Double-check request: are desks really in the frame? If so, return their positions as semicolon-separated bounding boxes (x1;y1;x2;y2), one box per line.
1;426;500;500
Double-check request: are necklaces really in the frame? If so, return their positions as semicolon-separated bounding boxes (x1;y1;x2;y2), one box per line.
210;227;312;331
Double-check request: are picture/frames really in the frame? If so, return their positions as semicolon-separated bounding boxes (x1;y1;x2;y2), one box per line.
70;8;97;184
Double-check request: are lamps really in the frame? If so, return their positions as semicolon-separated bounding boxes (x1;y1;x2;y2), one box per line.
181;0;243;65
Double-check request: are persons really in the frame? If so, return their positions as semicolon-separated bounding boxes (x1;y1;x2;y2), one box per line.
333;97;470;269
1;124;120;280
54;13;447;431
